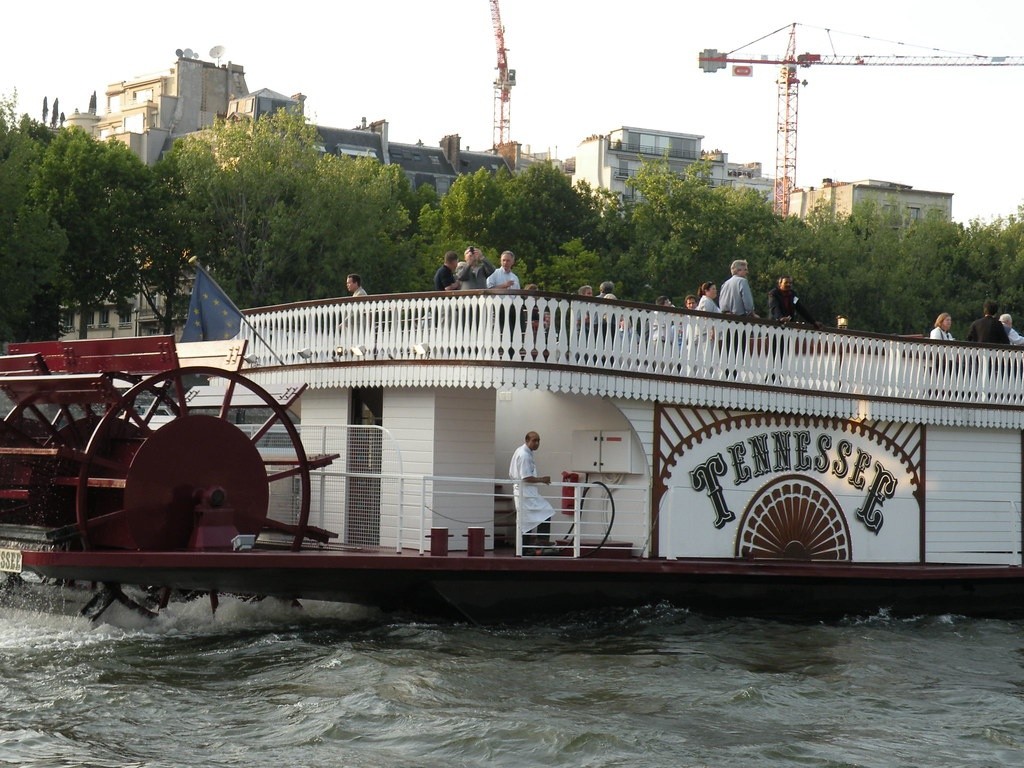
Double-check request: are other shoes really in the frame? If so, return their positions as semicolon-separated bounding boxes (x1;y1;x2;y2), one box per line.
536;539;555;546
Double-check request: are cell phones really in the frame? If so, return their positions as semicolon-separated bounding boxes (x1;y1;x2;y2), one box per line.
470;246;475;254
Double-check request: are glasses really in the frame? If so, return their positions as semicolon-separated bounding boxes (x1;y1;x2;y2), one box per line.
706;281;711;288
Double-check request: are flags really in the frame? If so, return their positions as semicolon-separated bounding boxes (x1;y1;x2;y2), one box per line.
179;265;245;344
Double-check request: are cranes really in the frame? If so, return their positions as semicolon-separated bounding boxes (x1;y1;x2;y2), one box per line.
483;0;517;166
699;21;1023;220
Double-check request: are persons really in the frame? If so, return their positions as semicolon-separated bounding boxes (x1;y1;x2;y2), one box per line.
965;302;1005;398
719;259;760;380
575;281;640;368
765;275;824;383
487;250;551;362
434;251;459;355
508;431;555;556
344;273;376;360
455;245;496;355
998;313;1024;345
652;281;721;374
929;312;954;397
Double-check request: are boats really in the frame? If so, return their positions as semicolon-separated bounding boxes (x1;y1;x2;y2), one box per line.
0;254;1023;627
